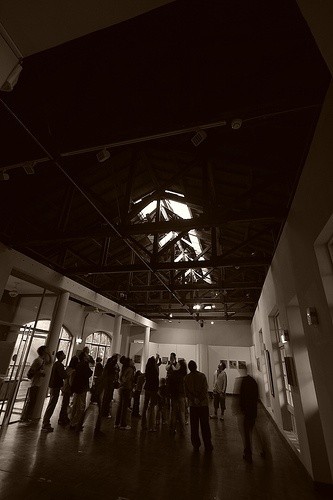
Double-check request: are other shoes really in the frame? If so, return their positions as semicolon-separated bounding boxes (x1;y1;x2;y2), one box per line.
220;416;224;420
41;424;54;432
210;413;218;419
18;421;30;427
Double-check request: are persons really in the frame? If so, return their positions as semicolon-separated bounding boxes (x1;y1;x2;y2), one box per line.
232;364;259;461
20;346;191;436
210;362;228;420
185;359;213;454
